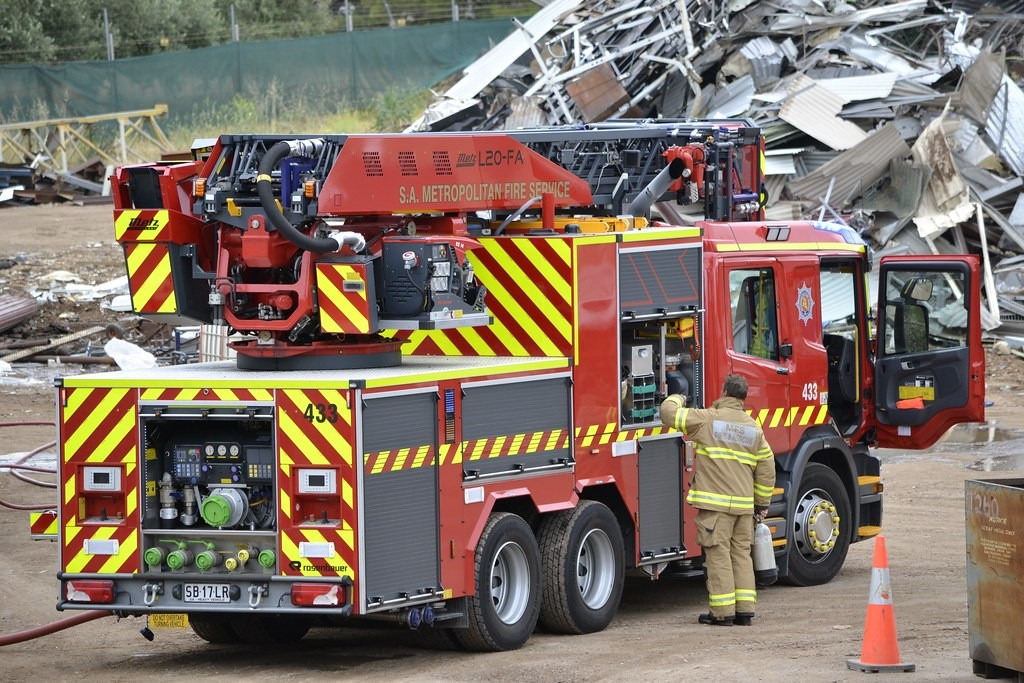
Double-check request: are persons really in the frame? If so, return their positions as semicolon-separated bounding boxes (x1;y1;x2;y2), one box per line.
659;374;776;626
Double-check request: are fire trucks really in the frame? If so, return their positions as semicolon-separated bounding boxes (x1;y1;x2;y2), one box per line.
29;117;986;654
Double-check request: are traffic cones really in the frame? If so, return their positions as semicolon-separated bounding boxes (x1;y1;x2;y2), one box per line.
846;535;915;674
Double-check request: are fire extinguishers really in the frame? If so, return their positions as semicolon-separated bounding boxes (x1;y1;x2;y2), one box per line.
752;508;779;586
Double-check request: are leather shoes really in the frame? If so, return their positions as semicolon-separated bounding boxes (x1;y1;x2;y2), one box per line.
698;613;753;627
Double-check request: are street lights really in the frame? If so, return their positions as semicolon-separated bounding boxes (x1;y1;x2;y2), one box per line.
339;0;355;32
